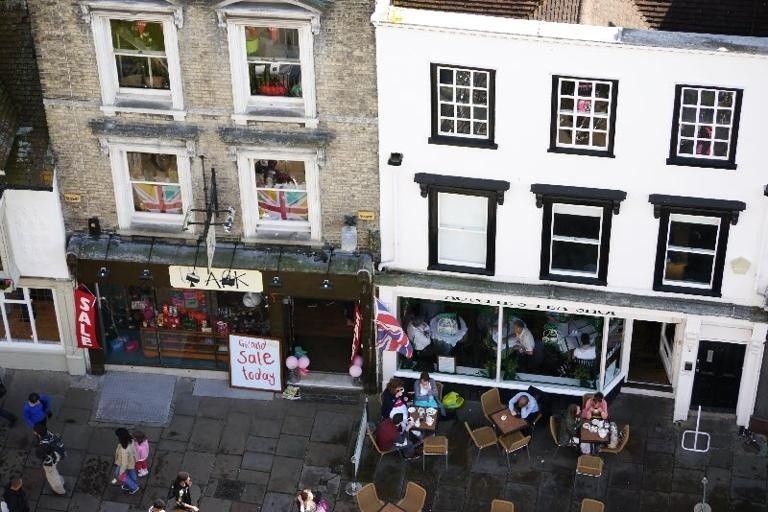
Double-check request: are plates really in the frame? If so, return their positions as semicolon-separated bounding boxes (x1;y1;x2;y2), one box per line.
407;405;437;416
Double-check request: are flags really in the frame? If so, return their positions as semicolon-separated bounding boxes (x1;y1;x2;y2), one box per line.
375;301;414;359
132;182;183;214
257;190;308;221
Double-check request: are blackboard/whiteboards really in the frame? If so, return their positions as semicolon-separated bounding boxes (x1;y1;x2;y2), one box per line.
227;332;284;393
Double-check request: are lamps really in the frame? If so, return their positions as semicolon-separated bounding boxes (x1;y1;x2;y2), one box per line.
96;231;336;291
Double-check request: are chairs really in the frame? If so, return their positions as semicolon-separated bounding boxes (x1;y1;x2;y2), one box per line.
350;381;633;511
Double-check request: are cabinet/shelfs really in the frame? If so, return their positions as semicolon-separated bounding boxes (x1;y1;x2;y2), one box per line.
139;325;159;360
155;327;216;363
213;333;230;365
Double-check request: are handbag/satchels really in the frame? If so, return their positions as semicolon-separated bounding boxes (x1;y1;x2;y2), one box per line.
167;479;177;500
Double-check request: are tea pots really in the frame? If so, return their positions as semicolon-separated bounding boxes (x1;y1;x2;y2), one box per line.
216;321;228;333
412;416;435;427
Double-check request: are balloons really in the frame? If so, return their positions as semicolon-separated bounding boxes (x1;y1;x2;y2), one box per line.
353;356;364;367
349;365;362;378
296;356;310;368
285;356;298;370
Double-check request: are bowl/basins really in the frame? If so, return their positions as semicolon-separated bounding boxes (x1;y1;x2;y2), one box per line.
246;35;258;56
144;76;168;89
669;262;688;279
583;418;610;438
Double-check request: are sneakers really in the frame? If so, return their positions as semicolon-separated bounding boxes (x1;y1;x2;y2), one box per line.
112;469;150;495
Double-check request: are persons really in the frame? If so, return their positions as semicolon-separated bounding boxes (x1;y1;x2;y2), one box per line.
295;487;330;512
133;429;149;477
582;391;608;421
381;377;408;417
0;378;18;430
148;499;166;512
511;319;535;357
507;390;539;450
32;422;68;495
555;403;582;448
0;475;31;512
413;370;438;439
571;331;597;367
406;315;438;362
23;392;54;442
172;471;200;512
546;310;557;323
112;427;140;495
375;413;421;461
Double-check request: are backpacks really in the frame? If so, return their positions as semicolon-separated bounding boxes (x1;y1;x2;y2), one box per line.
41;431;65;465
